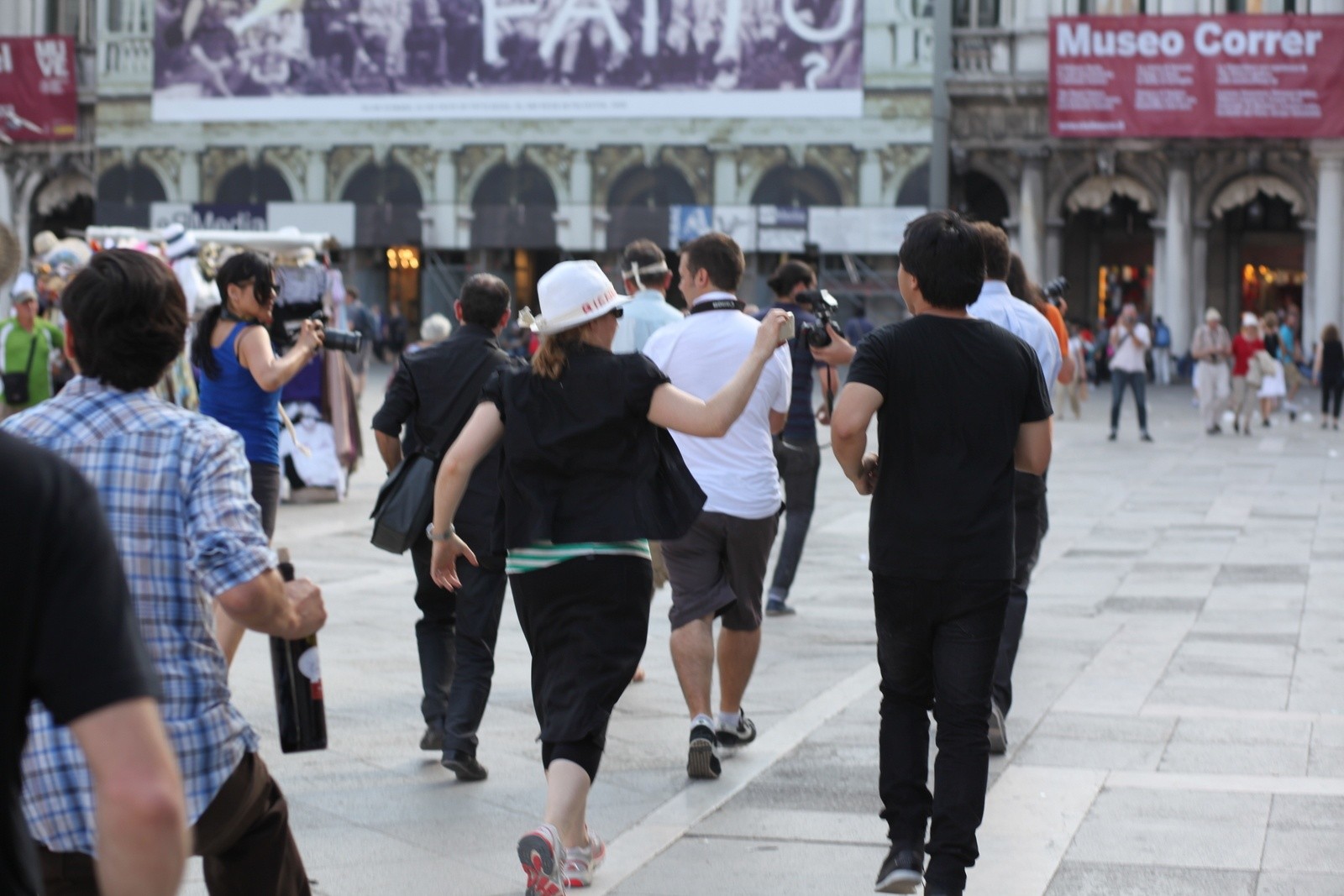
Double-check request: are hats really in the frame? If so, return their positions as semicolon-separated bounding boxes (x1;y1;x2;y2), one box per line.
531;260;632;334
420;312;451;344
9;270;38;305
1242;312;1258;326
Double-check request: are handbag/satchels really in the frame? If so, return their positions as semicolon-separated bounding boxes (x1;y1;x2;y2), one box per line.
369;450;436;555
3;371;29;403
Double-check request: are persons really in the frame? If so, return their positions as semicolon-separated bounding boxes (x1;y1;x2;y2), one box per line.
0;248;326;896
323;243;404;400
606;239;689;685
375;274;522;784
501;304;543;364
1311;325;1344;430
1107;302;1153;441
1054;313;1171;422
743;261;837;618
430;259;788;896
1190;298;1310;438
965;217;1070;756
846;308;872;344
830;206;1054;896
637;232;794;782
402;314;451;354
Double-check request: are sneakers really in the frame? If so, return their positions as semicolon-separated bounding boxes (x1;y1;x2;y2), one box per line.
518;830;564;896
420;731;444;749
715;709;755;749
687;726;721;778
560;831;605;886
441;749;486;779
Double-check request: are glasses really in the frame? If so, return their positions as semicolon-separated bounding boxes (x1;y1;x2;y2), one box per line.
612;308;624;319
237;279;280;298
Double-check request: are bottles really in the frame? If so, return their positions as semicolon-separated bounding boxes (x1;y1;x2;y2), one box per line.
270;561;329;752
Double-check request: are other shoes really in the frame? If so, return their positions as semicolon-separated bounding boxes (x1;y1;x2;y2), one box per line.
767;599;794;615
875;848;922;894
1141;435;1151;442
989;705;1007;755
1109;434;1116;441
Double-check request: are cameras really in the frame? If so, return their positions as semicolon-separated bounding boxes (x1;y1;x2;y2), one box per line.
798;289;843;349
779;311;795;341
1037;277;1069;305
308;310;361;353
